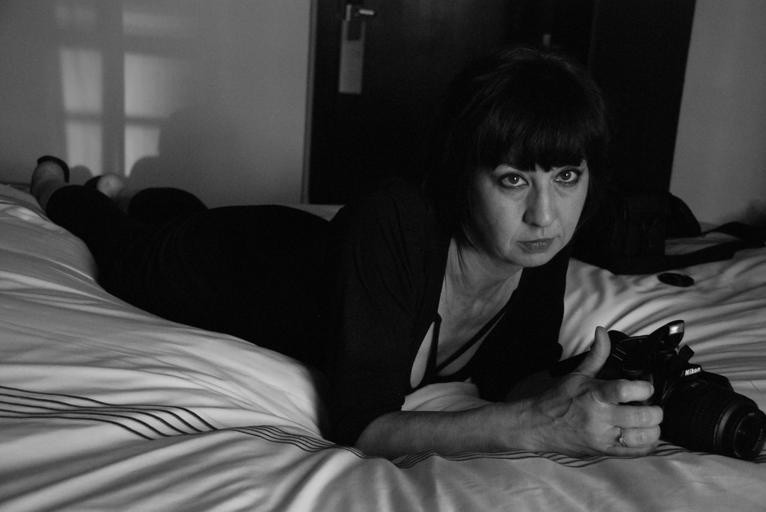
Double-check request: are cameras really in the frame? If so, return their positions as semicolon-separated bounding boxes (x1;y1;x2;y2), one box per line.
589;318;766;462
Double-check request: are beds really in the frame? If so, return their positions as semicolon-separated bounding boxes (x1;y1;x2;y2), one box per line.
0;205;766;511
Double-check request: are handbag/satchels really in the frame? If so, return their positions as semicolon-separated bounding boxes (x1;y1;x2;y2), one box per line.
564;180;700;276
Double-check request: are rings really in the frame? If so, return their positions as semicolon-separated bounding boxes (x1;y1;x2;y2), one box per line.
618;427;628;448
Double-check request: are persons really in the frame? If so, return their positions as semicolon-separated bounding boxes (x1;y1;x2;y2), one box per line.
28;45;665;460
